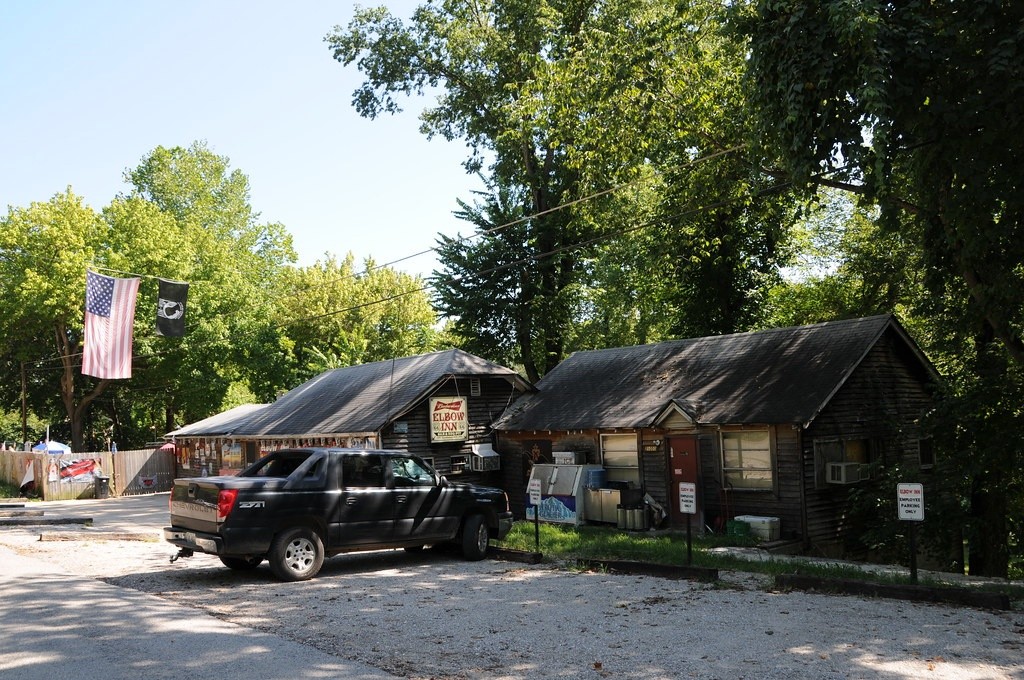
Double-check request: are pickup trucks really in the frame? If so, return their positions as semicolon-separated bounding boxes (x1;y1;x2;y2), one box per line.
165;443;512;582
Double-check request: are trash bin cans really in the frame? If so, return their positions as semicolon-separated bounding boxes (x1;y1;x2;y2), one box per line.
633;508;644;531
617;504;627;530
95;476;110;499
626;505;634;531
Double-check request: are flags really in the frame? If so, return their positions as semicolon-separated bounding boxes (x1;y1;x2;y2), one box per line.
156;277;189;335
81;270;140;380
